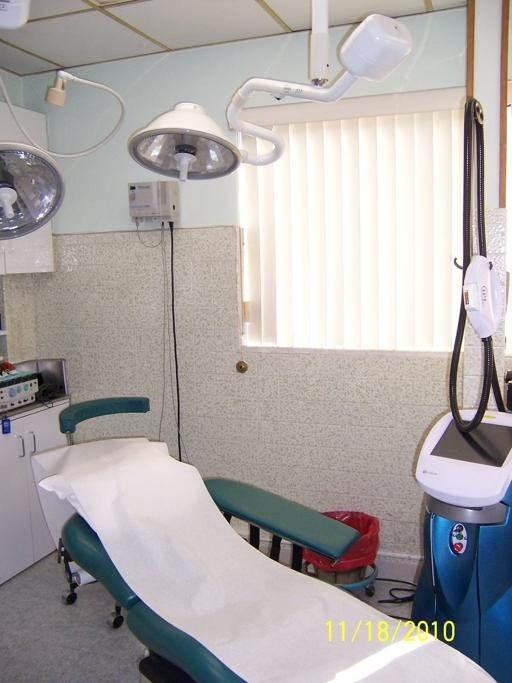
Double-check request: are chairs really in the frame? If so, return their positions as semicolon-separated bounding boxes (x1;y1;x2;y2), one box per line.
57;396;495;682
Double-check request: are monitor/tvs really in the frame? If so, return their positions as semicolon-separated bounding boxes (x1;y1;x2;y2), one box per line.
415;409;512;508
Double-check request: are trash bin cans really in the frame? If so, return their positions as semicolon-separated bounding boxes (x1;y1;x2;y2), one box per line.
302;511;380;597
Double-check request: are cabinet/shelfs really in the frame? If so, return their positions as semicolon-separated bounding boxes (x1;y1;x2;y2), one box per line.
0;402;71;588
0;101;56;276
